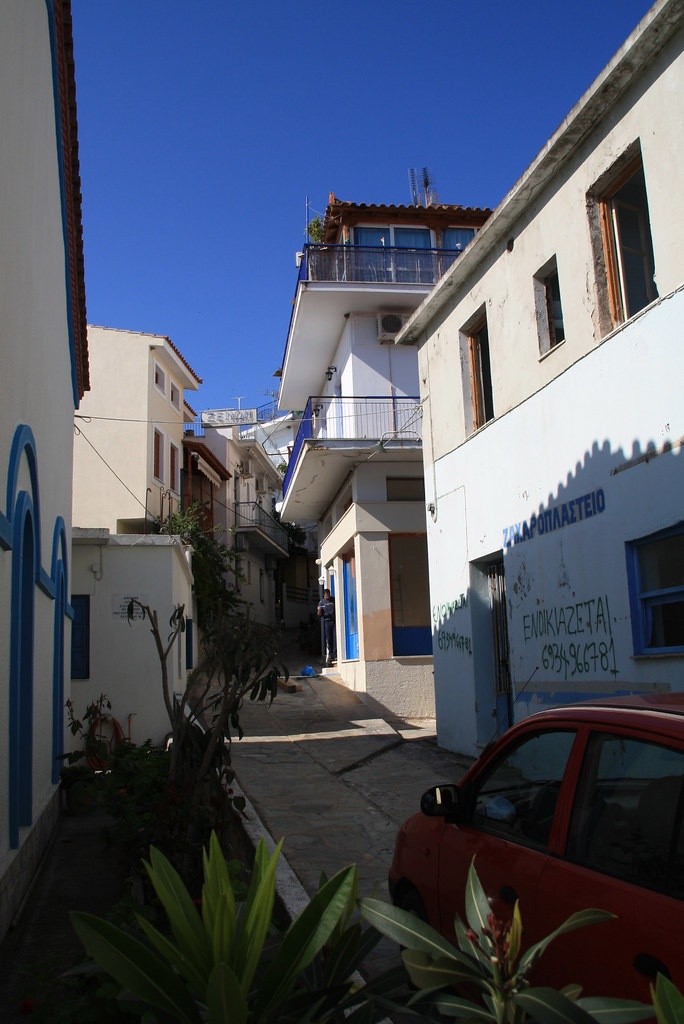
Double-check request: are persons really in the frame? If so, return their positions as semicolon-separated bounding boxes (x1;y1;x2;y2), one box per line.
317;588;336;666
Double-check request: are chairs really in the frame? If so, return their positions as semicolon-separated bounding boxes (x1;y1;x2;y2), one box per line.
619;775;684;899
528;778;562;844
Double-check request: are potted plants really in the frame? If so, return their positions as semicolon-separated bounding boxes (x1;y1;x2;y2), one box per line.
55;693;112;815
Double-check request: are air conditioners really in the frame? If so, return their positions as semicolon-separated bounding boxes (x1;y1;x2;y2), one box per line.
236;533;248;552
265;552;276;570
376;312;410;341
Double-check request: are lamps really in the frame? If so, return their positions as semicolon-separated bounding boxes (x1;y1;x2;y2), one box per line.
313;404;323;417
325;365;337;381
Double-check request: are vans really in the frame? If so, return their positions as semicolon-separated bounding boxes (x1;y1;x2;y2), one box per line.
386;689;683;1024
